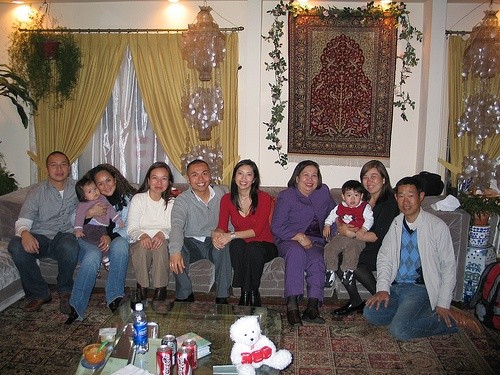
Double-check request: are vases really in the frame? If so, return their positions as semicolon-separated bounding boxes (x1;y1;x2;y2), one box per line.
464;225;497;303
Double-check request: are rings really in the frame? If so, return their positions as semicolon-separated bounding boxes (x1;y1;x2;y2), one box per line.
171;263;173;266
107;245;109;247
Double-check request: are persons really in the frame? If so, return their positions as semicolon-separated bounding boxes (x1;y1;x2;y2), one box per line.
168;159;234;304
211;160;278;306
363;177;465;339
74;179;124;279
59;164;138;324
126;161;176;301
270;160;339;326
334;160;400;315
323;180;374;287
8;151;79;315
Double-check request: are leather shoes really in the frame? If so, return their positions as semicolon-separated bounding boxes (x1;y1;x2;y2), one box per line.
60;293;69;302
168;293;194;311
333;300;366;316
23;293;52;312
215;297;230;314
249;289;262;307
238;290;250;306
448;305;484;338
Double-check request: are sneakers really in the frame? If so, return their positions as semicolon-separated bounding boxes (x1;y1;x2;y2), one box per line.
96;270;102;279
100;256;110;271
324;270;337;288
341;269;353;287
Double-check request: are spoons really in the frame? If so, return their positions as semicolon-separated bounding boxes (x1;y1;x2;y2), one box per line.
96;340;109;353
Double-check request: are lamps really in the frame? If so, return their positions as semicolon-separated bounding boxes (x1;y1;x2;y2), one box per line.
180;0;228;188
453;1;500;191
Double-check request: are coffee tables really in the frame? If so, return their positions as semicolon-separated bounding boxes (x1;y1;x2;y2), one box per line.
74;300;284;375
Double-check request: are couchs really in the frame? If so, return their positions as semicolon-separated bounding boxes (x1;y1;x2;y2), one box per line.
0;183;472;312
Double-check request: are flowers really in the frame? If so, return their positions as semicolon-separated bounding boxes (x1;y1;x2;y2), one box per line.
261;0;424;170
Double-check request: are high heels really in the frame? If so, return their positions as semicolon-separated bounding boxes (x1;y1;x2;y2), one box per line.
109;298;135;315
60;300;78;325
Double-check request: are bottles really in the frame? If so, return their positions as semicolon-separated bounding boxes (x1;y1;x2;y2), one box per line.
133;303;149;354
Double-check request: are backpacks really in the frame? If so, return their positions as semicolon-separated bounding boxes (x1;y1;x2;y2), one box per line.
469;262;500;331
392;171;444;196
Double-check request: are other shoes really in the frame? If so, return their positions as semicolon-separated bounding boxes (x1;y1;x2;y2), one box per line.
286;303;302;326
302;306;325;324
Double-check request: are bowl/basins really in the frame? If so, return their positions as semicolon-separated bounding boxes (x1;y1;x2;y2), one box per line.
84;344;106;364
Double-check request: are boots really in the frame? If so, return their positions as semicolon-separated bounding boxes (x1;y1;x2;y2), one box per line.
134;283;148;312
151;288;168;316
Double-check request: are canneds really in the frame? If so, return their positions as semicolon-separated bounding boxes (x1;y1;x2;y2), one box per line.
181;338;198;370
175;347;194;375
156;345;172;375
161;334;177;365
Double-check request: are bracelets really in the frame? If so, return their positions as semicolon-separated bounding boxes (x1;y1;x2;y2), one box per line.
20;230;27;236
298;234;306;243
353;231;356;240
230;231;236;240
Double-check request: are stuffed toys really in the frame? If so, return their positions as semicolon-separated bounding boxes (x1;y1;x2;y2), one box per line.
230;316;292;375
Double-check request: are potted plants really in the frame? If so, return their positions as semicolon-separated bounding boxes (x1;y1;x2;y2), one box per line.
4;0;86;117
458;192;500;226
